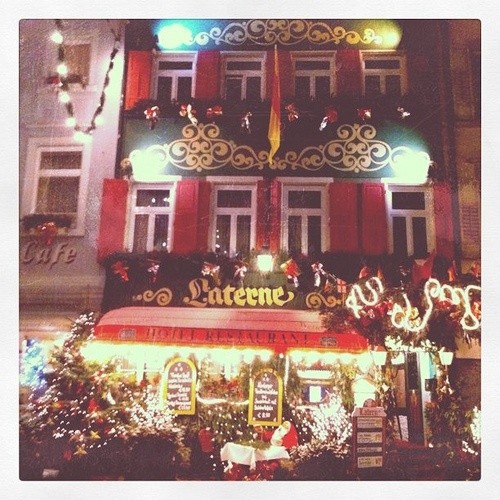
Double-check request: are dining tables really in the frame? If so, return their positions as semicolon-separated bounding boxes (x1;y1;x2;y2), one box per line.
220;441;290;481
